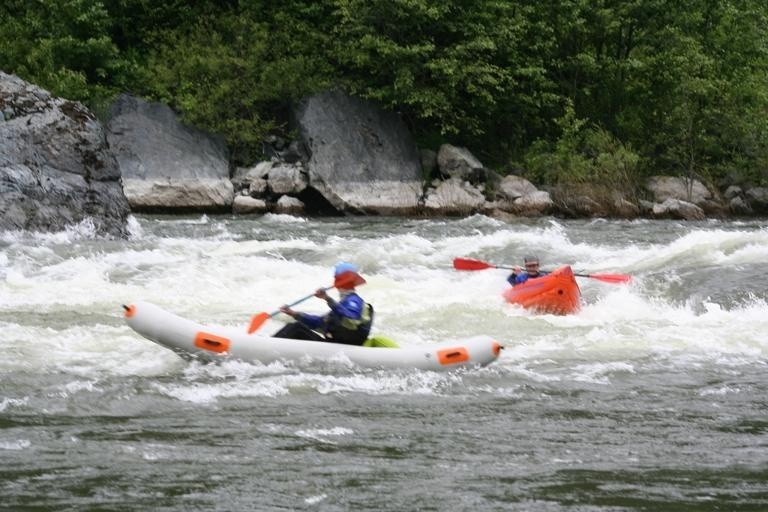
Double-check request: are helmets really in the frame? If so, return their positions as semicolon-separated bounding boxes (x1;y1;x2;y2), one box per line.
334;261;358;279
524;254;540;266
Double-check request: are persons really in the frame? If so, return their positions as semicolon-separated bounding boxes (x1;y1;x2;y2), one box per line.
507;255;550;287
270;261;373;344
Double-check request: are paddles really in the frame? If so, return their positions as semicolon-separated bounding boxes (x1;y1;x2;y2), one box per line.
454;257;633;283
246;270;366;334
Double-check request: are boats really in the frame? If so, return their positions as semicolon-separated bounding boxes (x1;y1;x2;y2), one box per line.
501;264;586;320
121;298;501;377
